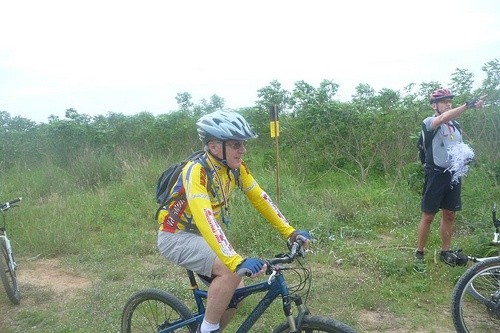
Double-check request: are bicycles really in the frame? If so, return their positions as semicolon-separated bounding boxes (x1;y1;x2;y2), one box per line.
450;207;500;333
0;198;22;305
121;239;357;333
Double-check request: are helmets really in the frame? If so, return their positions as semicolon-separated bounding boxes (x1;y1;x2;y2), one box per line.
431;88;455;99
196;110;254;145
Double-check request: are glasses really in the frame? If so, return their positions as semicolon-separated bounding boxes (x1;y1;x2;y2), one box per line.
228;141;248;149
440;99;452;103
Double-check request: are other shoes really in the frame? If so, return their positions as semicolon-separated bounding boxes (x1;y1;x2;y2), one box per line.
195;324;221;333
439;250;452;261
413;251;425;273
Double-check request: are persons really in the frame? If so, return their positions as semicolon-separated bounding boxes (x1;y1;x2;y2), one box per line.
413;88;488;274
157;110;311;333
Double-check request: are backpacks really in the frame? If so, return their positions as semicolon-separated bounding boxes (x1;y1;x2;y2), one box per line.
417;117;461;166
156;149;213;203
